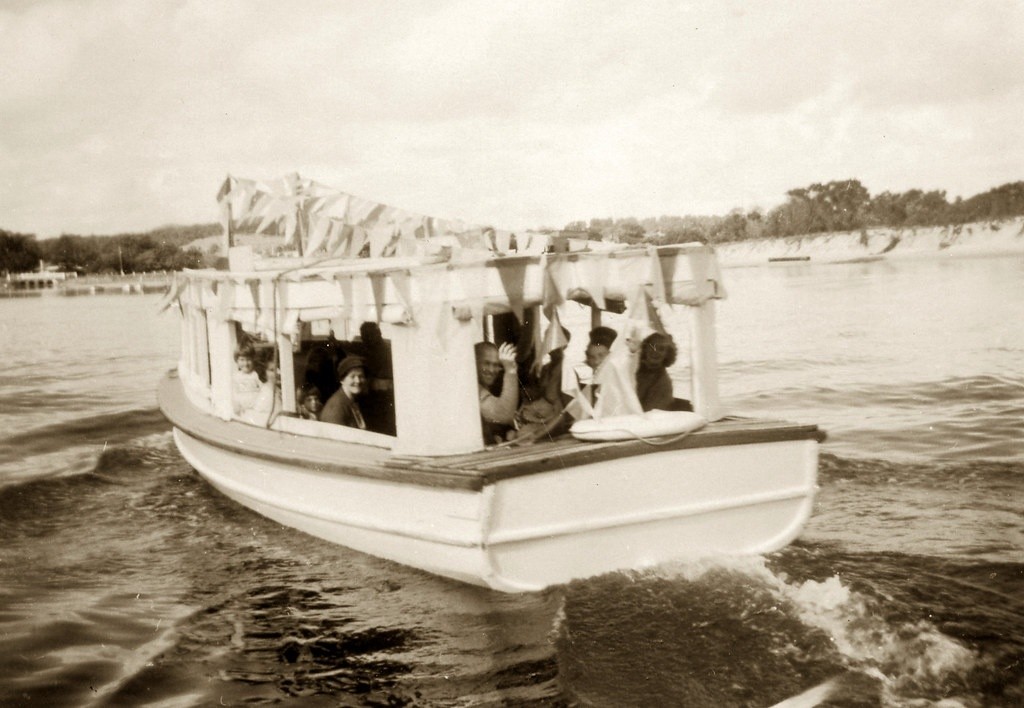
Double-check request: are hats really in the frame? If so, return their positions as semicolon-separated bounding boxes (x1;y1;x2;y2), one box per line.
338;356;363;376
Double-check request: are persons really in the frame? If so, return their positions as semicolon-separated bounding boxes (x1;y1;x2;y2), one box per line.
235;317;681;443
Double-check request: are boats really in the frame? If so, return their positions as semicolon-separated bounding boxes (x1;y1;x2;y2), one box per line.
153;241;830;597
